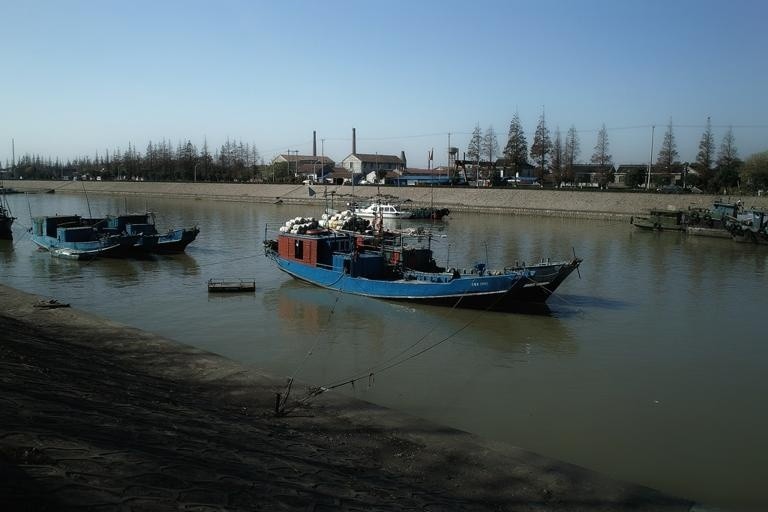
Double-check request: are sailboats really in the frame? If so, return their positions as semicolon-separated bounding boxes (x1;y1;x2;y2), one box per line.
262;138;584;301
0;185;17;240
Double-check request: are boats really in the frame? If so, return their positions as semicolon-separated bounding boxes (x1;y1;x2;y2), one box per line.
411;207;451;220
629;201;768;244
31;213;120;258
105;206;200;255
348;202;414;219
